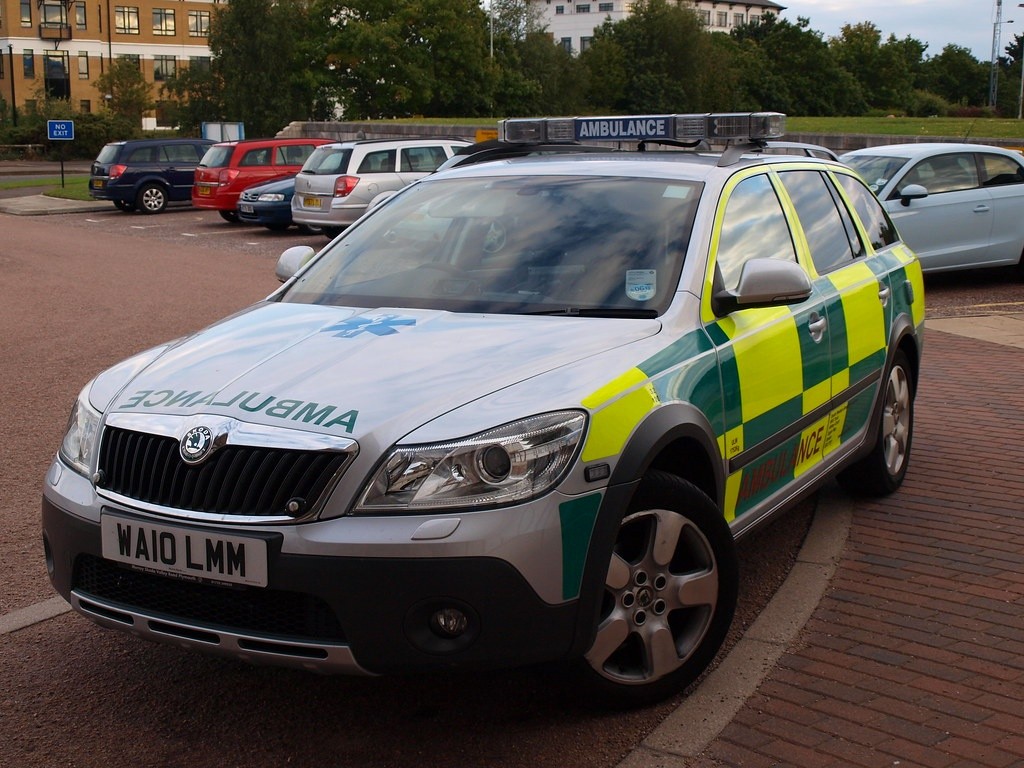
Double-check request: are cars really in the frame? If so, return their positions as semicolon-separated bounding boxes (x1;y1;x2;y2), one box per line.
836;142;1022;282
37;109;927;710
236;171;322;237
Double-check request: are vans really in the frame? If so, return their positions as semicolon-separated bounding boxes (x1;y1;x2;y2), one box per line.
87;137;220;215
290;136;476;243
192;137;339;223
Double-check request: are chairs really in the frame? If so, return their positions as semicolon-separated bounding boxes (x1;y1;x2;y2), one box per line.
933;165;970;185
248;153;258;165
886;164;920;186
291;147;304;165
410;155;424;171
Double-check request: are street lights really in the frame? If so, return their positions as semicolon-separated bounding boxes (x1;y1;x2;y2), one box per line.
989;19;1015;115
